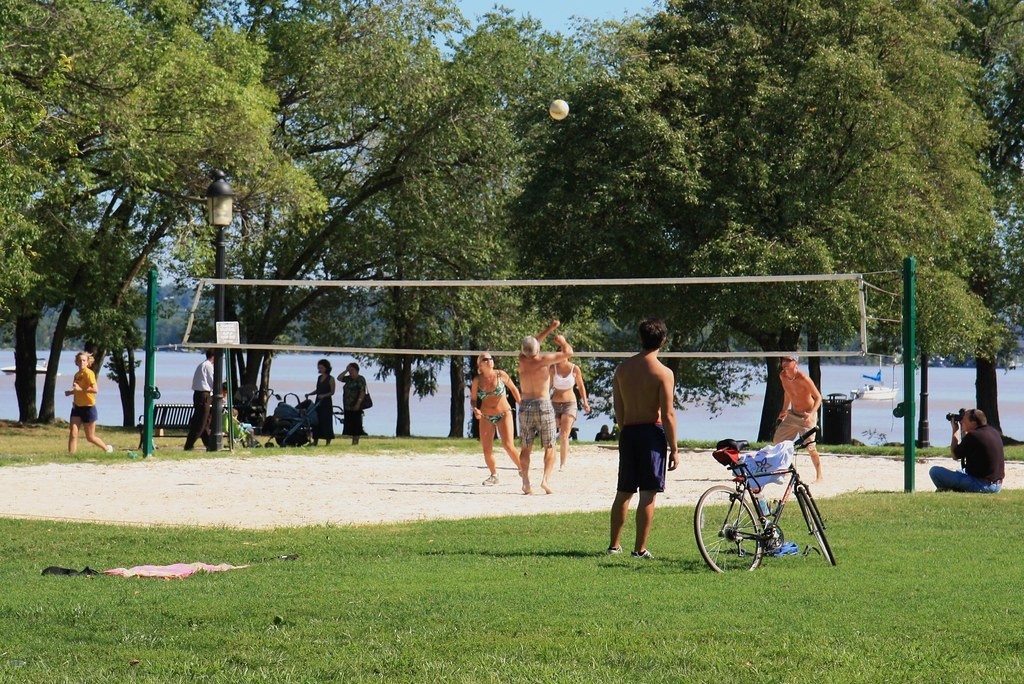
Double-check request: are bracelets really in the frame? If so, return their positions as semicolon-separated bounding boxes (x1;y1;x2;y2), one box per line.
358;399;362;402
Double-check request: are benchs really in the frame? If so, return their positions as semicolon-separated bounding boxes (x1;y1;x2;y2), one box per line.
137;404;253;450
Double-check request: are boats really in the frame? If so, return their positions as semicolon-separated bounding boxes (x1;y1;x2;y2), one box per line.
850;356;900;401
0;357;48;375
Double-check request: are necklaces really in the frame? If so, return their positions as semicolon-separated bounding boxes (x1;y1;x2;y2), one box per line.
787;376;795;382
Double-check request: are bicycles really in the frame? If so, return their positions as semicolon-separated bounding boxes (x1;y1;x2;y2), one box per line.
693;426;837;573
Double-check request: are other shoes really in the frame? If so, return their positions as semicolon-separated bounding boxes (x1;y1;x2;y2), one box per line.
482;474;500;486
518;471;522;477
106;445;113;452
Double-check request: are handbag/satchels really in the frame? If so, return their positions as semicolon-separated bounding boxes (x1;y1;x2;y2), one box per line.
360;394;373;409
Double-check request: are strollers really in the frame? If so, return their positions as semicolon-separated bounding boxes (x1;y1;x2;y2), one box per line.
241;388;282;435
262;392;322;449
222;398;263;448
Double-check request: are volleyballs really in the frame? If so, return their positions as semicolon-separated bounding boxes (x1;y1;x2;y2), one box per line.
549;99;569;121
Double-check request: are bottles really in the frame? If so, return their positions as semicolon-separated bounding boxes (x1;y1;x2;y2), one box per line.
754;488;769;516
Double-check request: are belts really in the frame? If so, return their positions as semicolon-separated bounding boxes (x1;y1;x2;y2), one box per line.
974;475;1003;484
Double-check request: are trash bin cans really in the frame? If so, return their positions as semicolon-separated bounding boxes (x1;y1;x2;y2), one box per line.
816;393;855;444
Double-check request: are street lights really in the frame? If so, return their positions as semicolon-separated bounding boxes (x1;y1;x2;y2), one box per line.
204;168;236;453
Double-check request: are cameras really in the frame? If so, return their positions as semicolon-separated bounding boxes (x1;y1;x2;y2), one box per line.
946;408;966;421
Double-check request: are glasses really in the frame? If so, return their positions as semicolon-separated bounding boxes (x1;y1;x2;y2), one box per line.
479;358;493;365
782;358;795;362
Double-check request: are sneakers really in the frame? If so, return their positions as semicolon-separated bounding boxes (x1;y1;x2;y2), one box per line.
607;544;622;554
630;549;654;558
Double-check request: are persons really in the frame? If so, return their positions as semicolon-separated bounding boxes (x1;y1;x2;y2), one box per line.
184;349;227;452
610;417;620;440
772;356;823;484
308;359;336;447
233;409;254;434
595;425;610;441
471;353;521;486
65;352;113;454
550;347;590;471
929;409;1006;494
606;318;680;557
337;363;368;444
519;320;574;494
